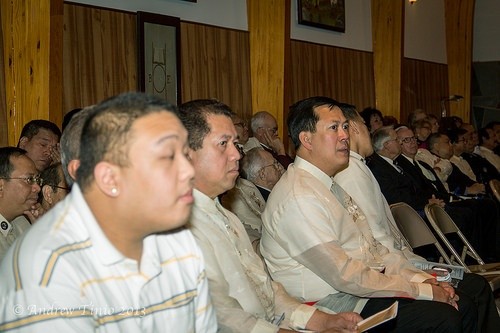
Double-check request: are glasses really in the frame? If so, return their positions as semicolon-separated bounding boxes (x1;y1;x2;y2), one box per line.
402;136;415;143
0;176;43;187
234;122;246;127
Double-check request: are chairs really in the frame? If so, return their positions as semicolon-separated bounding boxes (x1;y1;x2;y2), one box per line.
488;179;500;201
389;201;500;316
424;202;500;274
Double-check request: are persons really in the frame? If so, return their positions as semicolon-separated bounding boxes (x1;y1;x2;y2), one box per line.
0;92;500;333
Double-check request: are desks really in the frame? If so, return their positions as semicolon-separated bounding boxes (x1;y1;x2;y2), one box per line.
444;201;500;266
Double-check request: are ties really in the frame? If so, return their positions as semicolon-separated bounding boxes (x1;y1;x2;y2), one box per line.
393;160;405;175
413;159;422;175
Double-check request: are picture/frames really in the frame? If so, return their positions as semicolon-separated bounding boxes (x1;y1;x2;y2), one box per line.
178;0;199;5
296;0;346;33
137;10;183;110
